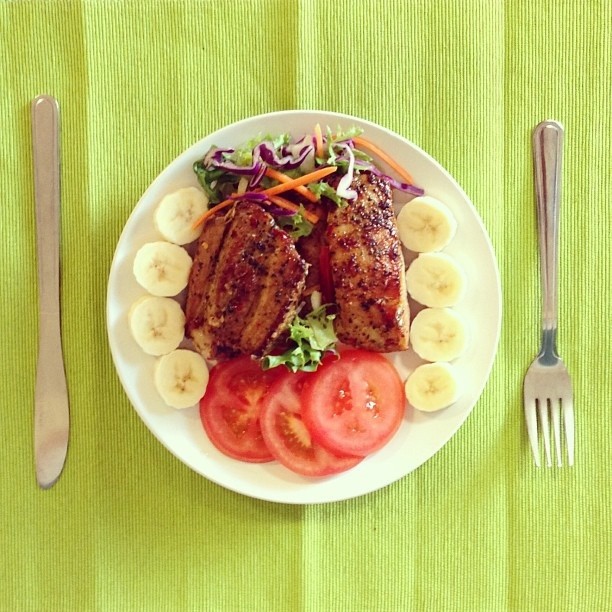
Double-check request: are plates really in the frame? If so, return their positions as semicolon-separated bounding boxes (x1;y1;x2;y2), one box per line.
106;109;503;505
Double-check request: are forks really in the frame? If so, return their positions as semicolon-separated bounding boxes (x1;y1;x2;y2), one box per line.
524;121;577;471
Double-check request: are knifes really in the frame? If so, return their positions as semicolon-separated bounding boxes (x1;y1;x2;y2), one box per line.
30;94;69;486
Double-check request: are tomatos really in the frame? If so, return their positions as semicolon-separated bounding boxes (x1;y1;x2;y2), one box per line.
198;353;279;464
259;359;366;478
299;346;405;459
